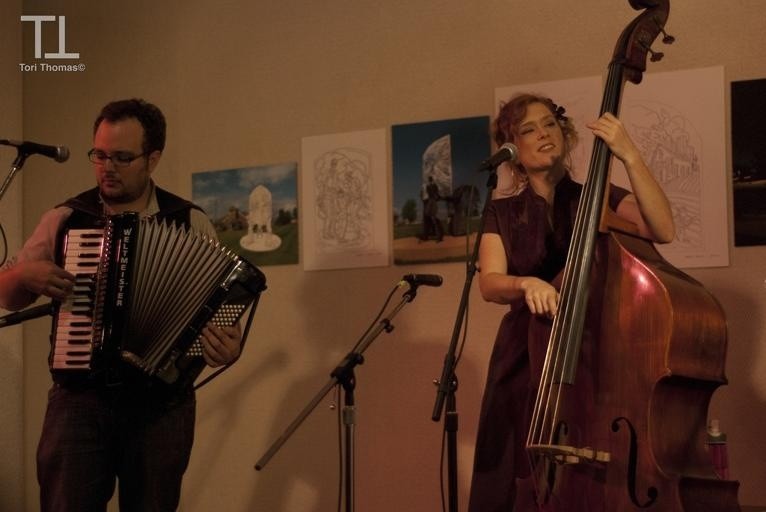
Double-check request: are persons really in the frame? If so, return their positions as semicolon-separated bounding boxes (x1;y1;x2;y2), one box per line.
468;93;675;512
0;98;242;512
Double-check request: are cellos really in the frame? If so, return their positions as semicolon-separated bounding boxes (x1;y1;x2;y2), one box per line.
467;2;739;510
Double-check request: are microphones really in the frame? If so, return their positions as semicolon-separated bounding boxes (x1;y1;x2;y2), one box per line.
486;142;518;171
404;274;443;287
7;138;70;162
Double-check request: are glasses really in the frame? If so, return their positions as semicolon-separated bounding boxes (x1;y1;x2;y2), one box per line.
88;147;147;167
